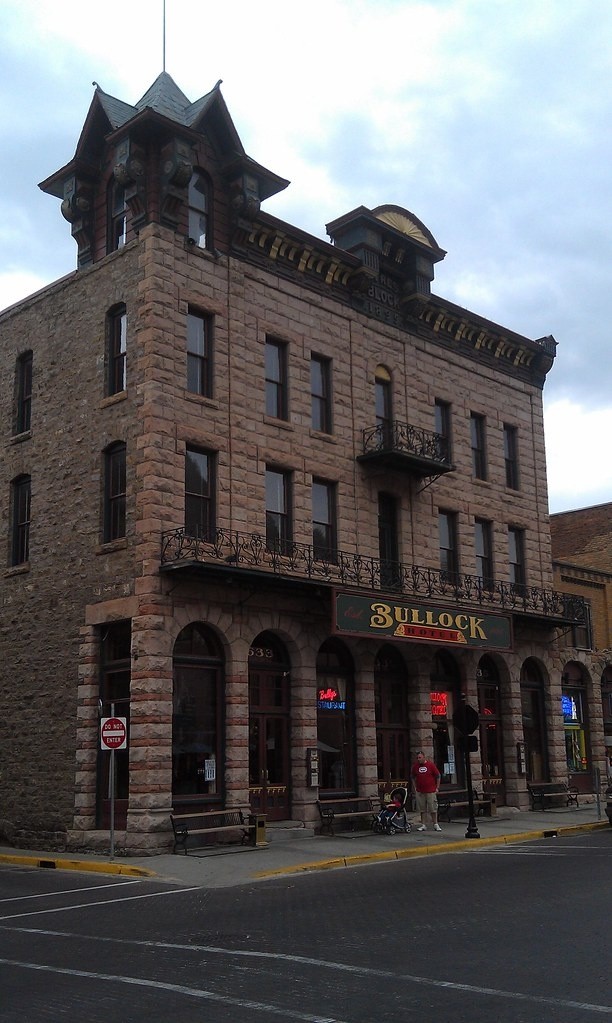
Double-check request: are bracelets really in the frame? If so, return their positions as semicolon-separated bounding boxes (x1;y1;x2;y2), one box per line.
437;787;440;790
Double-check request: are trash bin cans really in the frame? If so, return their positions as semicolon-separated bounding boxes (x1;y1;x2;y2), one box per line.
247;814;268;846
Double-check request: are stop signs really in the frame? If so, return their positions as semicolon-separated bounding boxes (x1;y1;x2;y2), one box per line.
100;718;127;750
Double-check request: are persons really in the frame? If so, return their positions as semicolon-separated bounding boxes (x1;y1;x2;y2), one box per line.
571;696;578;719
410;751;442;831
376;793;403;823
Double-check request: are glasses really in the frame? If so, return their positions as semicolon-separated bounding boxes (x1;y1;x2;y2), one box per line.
417;751;423;755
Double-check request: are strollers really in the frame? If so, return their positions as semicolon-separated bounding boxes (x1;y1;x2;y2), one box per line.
374;787;411;835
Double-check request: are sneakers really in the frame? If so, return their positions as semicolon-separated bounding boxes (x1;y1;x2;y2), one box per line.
416;823;427;832
434;823;442;831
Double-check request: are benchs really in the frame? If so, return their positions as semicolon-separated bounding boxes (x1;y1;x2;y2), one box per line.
527;781;580;812
437;790;490;824
170;809;256;857
317;797;381;836
